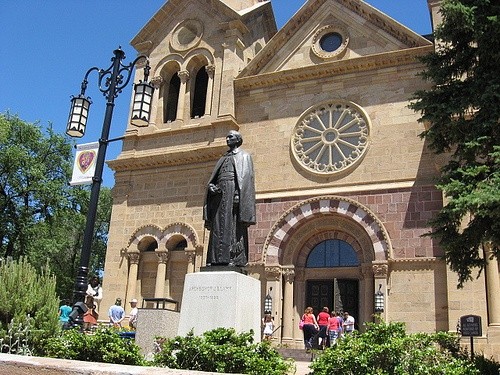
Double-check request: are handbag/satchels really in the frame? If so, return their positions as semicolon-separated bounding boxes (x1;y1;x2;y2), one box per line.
299;320;304;330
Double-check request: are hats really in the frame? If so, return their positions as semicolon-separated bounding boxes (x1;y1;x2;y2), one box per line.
129;298;138;302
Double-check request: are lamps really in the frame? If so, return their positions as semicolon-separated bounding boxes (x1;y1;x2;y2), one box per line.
374;283;385;314
263;286;273;315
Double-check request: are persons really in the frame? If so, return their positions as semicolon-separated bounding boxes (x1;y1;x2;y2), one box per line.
200;130;257;272
262;307;355;353
84;275;103;314
59;295;138;331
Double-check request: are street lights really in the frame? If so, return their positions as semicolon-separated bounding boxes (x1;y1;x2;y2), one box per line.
65;45;155;305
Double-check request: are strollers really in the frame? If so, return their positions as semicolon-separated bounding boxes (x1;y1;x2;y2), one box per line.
305;326;327;352
